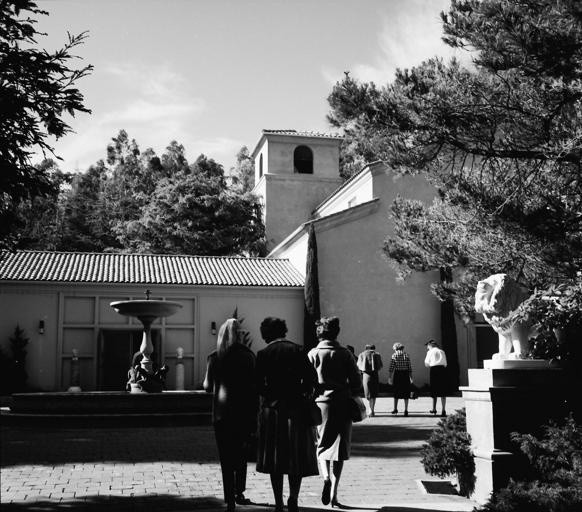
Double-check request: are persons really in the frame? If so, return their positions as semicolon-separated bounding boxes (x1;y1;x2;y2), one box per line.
387;341;415;415
250;315;322;511
200;316;258;505
346;344;358;363
356;344;385;416
124;351;171;392
423;339;448;418
305;316;366;508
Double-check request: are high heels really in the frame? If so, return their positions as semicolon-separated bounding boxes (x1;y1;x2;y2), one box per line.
392;409;408;416
322;480;342;507
430;410;446;416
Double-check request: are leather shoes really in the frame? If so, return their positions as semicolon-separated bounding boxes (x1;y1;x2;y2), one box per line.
228;493;250;512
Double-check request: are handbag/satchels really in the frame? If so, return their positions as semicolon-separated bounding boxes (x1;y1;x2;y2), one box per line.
409;391;418;399
352;397;367;423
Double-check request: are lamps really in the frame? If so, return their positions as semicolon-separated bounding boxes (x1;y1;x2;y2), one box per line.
39;320;45;334
211;321;216;335
